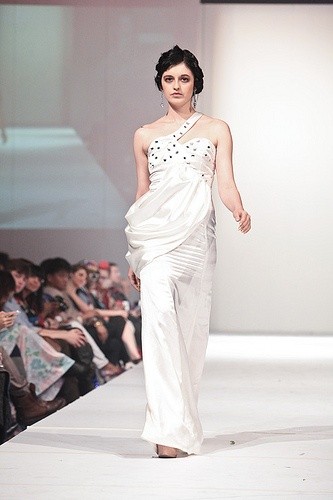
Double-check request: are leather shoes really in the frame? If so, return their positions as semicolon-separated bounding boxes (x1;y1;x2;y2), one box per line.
72;363;90;375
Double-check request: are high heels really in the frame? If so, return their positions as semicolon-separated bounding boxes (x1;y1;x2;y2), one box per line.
156;444;178;458
99;368;126;384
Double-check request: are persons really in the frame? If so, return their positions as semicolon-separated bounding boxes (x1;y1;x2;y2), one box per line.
0;251;143;432
124;45;252;458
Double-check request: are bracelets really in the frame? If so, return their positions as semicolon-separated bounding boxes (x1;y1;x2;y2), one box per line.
93;319;103;328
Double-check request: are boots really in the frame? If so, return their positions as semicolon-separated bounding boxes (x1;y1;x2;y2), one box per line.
9;383;66;426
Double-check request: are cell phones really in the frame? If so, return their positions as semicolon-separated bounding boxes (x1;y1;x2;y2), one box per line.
9;310;20;317
